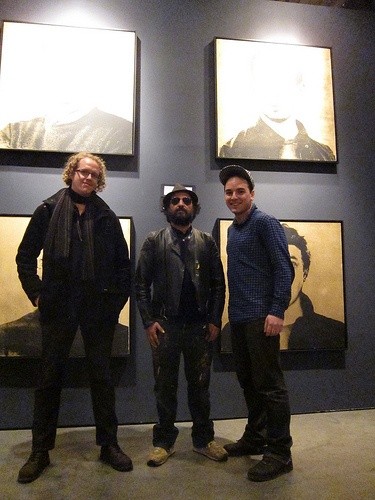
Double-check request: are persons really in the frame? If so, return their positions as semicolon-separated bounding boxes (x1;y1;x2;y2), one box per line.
137;182;229;467
219;165;293;482
13;153;133;483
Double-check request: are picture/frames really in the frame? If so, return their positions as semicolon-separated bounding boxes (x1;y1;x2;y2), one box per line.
213;36;339;164
0;20;138;158
217;218;349;357
159;184;195;212
0;214;135;360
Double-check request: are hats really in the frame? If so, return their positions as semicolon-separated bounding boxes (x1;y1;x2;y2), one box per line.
162;184;198;206
219;165;256;190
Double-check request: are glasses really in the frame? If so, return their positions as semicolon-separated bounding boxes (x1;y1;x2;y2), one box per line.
170;197;192;206
73;168;99;181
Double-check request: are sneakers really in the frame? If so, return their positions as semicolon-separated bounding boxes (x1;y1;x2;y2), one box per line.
247;457;293;482
100;443;132;470
18;450;50;481
193;440;228;460
223;440;263;456
147;445;175;466
